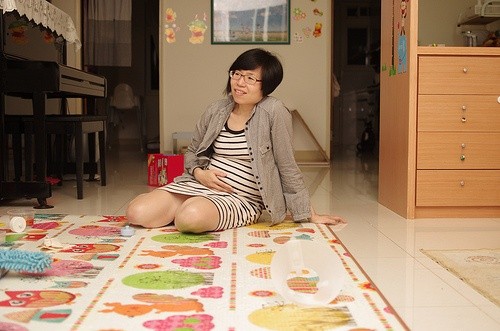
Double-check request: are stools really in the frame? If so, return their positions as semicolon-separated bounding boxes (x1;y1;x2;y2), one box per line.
25;114;107;200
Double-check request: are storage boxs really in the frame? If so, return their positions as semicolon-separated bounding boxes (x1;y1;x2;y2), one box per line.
148;154;185;187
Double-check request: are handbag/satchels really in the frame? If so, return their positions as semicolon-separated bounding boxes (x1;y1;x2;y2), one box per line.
158;154;185;186
148;154;163;187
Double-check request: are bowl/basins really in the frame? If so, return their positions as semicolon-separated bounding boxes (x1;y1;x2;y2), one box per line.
8;209;35;227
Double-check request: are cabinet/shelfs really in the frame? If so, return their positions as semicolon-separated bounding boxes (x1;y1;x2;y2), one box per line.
378;0;500;218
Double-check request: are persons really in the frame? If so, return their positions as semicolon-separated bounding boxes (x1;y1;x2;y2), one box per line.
126;48;346;233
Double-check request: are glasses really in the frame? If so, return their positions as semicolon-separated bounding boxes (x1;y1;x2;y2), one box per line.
228;70;265;85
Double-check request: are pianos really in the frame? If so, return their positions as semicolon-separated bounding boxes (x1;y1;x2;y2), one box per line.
0;0;107;209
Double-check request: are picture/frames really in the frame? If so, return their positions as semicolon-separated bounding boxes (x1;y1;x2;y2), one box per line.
209;0;292;46
